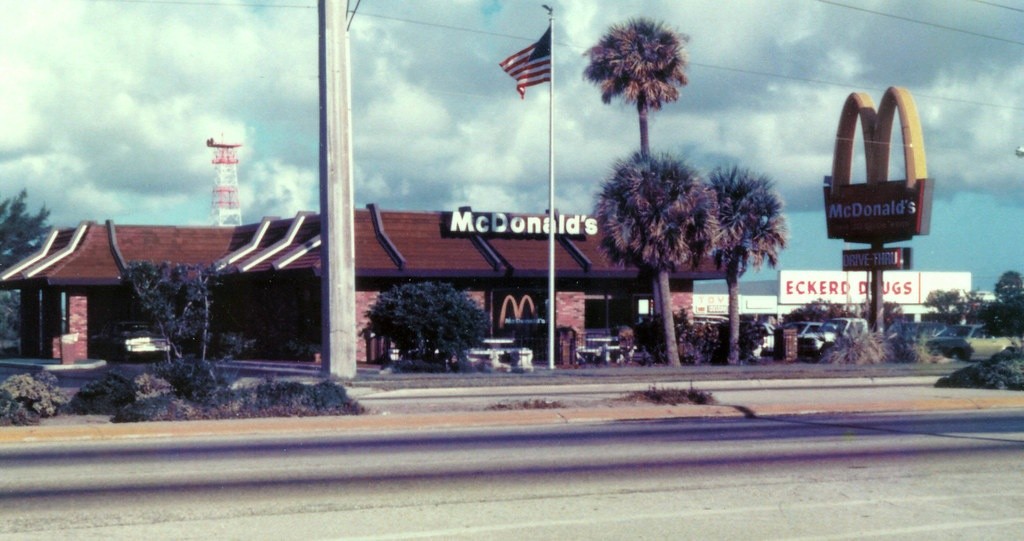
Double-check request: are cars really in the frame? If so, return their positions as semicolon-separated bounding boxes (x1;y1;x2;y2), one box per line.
928;322;1024;365
884;320;948;355
573;335;657;366
87;319;170;366
694;310;871;358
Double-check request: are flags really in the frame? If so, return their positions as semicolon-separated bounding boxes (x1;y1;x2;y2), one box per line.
499;24;552;99
